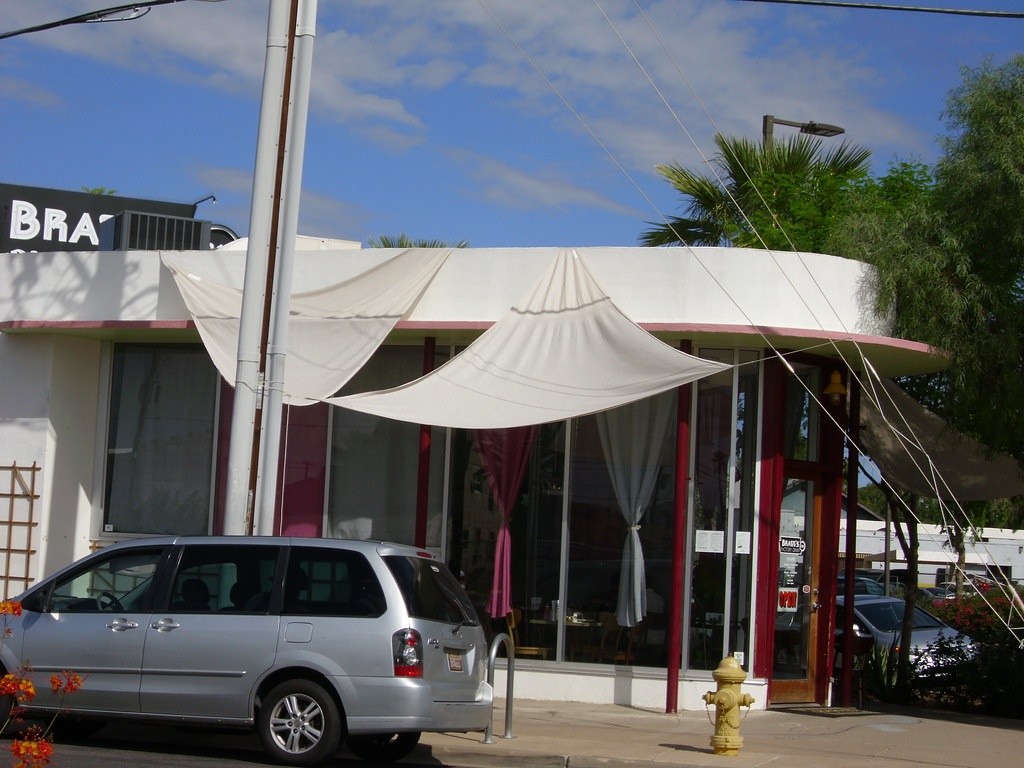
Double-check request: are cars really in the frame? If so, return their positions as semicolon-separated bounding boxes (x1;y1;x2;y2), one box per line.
793;562;980;605
773;592;995;706
0;535;494;768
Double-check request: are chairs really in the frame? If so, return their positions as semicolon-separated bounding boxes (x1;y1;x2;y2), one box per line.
170;579;238;609
834;633;875;710
505;608;667;665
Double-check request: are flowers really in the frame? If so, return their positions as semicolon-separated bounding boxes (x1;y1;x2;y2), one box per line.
0;601;84;768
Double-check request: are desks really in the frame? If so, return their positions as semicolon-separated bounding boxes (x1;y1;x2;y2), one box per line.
529;620;603;626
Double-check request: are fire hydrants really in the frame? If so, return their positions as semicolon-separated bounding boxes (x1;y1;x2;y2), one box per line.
701;653;756;755
